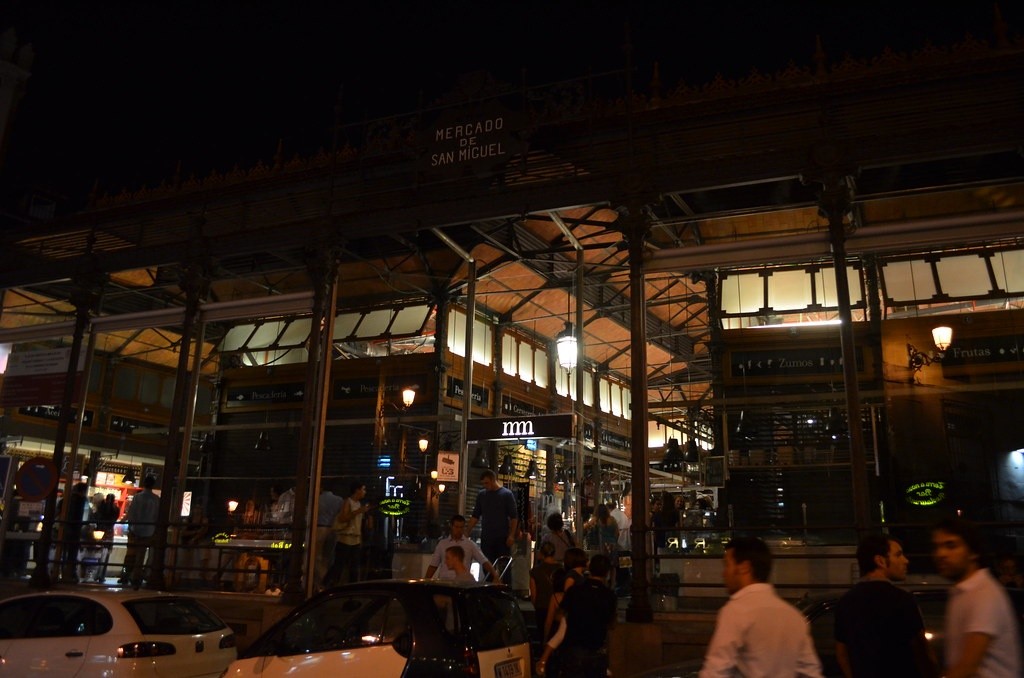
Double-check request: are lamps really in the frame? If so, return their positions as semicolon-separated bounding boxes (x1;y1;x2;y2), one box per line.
431;483;446;498
499;289;516;475
471;278;492;470
122;454;136;485
554;288;579;377
663;278;685;462
683;273;700;462
903;326;954;384
379;389;415;418
522;318;542;479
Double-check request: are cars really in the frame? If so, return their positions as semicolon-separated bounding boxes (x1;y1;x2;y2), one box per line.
221;576;533;678
0;587;240;678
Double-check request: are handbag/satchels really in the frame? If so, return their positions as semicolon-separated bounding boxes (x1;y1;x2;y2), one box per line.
322;533;336;556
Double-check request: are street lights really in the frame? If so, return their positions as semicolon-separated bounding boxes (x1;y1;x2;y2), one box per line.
556;319;577;414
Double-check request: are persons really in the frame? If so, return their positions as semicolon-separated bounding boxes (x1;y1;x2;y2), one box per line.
0;475;161;585
931;524;1024;678
527;511;619;678
425;515;502;584
465;469;519;589
834;533;942;678
266;468;372;593
699;537;824;678
444;546;478;584
579;489;716;590
